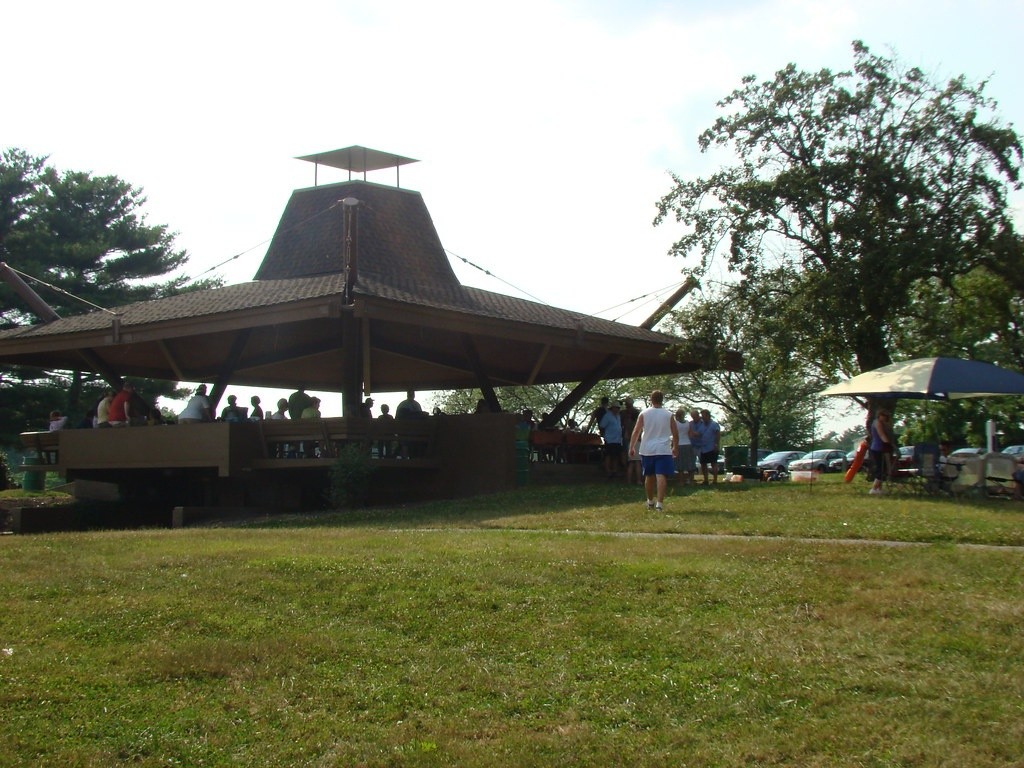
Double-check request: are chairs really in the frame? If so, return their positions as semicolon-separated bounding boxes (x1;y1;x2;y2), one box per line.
909;442;963;496
885;458;918;496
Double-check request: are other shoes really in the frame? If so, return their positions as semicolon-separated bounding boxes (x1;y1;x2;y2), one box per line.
656;505;661;511
648;503;654;507
868;488;882;495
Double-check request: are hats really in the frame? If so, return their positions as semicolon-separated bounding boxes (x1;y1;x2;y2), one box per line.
601;397;608;401
196;385;206;391
619;405;626;411
611;401;621;408
626;398;633;405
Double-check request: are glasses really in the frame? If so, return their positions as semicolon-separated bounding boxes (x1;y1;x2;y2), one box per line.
602;402;607;404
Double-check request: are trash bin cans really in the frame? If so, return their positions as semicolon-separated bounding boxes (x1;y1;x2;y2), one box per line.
723;445;749;473
22;457;47;491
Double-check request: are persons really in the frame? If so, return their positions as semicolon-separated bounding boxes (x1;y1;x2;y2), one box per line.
937;442;951;470
629;390;678;511
673;409;720;488
362;390;422;459
50;410;67;431
524;409;582;463
585;396;645;487
868;409;899;495
474;399;491;415
77;382;322;459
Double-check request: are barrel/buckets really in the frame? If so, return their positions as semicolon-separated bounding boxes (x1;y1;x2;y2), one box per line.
22;457;46;491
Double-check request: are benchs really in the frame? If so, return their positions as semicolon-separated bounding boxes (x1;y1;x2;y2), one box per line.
20;431;60;465
529;431;601;463
257;420;437;459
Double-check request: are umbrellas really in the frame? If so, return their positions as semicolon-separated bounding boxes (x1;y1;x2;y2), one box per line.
819;357;1024;500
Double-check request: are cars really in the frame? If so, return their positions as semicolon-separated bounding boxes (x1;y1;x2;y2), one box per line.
749;449;774;462
1001;445;1024;464
696;454;725;475
846;450;869;466
788;449;848;473
893;446;915;469
947;448;987;454
757;451;808;474
